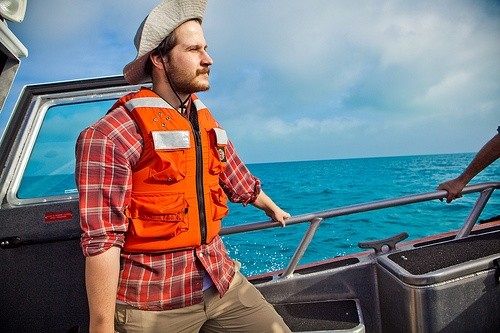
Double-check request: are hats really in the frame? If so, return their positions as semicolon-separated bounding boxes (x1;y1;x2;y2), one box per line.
123;0;208;85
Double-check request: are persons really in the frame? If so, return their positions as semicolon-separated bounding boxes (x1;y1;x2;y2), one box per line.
436;125;500;203
74;5;294;332
